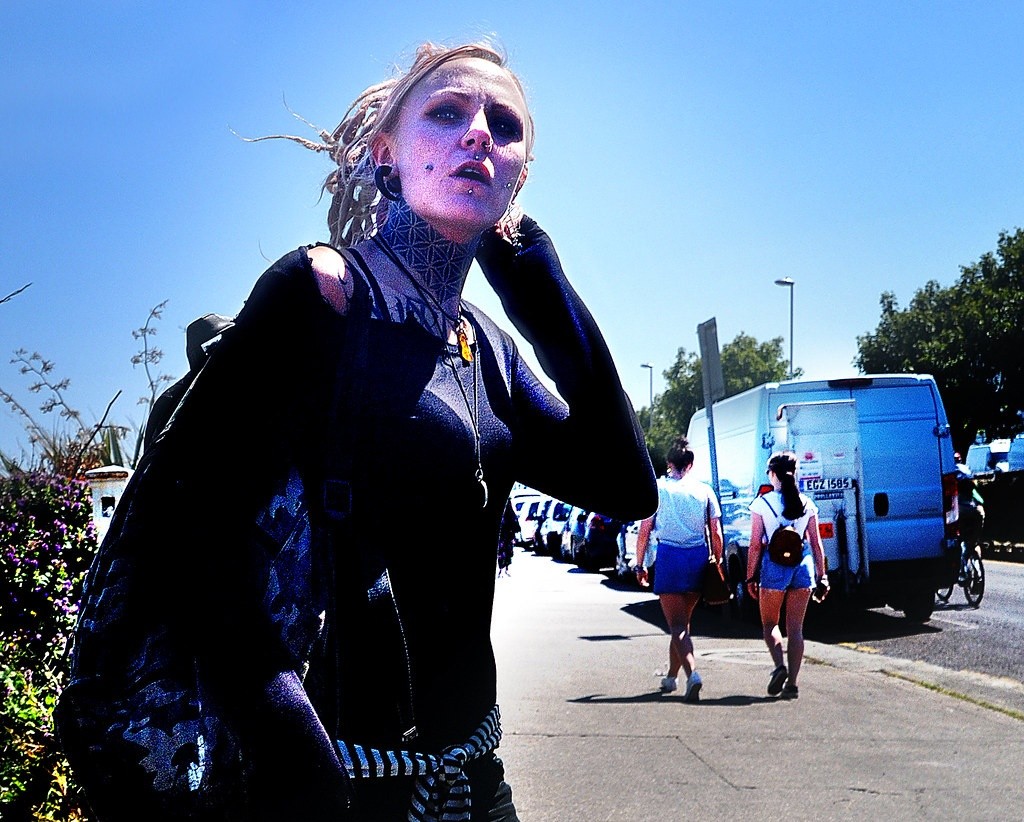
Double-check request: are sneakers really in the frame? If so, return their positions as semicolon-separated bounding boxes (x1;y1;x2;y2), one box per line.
780;681;799;699
767;665;789;695
685;672;703;702
661;677;678;690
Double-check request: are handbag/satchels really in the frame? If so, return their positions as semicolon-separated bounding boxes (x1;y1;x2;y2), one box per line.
704;555;731;600
512;517;521;533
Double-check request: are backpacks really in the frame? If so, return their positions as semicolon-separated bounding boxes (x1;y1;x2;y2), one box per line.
761;496;808;566
55;242;374;822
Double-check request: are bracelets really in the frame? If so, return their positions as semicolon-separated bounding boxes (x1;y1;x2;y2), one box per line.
815;576;827;582
636;565;645;573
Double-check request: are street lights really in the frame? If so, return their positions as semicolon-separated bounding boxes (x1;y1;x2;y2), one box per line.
775;278;795;382
640;364;653;410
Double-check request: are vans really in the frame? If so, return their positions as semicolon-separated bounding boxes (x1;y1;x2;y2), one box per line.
673;373;960;621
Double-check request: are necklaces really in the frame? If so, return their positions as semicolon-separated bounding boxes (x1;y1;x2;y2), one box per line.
372;234;490;511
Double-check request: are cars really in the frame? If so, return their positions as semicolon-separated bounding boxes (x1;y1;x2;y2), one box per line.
510;491;658;581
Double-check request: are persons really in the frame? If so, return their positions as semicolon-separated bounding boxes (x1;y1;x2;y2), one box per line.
495;495;520;581
747;450;830;701
210;43;658;822
951;450;984;559
634;438;723;703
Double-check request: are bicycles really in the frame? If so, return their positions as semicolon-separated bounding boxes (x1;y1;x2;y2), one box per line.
936;521;984;608
